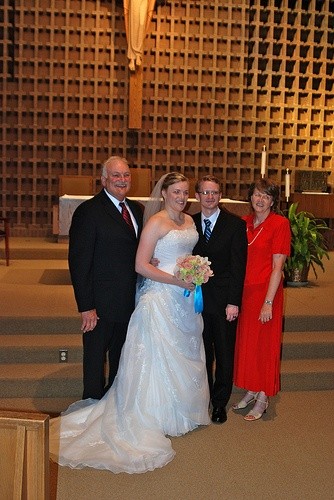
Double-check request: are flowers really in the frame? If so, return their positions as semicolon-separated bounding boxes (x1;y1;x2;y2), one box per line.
174;253;215;286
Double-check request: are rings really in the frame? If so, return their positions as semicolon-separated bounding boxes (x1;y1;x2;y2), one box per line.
233;316;236;318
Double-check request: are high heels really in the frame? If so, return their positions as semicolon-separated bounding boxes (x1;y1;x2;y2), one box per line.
232;392;270;421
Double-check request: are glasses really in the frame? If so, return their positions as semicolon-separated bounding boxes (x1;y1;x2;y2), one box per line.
196;190;221;195
253;192;272;200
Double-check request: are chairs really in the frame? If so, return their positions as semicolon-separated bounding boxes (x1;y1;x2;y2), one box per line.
126;167;152;197
52;173;97;235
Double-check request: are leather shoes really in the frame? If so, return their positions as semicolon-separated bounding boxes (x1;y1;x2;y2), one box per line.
211;406;227;425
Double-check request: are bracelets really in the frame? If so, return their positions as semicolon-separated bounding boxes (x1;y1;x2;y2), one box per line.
264;300;272;305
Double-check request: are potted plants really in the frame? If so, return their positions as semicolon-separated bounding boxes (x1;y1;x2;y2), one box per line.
283;200;334;284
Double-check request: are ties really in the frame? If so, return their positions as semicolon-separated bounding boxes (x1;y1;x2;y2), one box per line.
204;219;212;248
119;202;136;239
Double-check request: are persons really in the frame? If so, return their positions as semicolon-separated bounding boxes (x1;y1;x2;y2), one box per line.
67;156;147;399
150;174;247;424
105;171;213;438
231;179;291;420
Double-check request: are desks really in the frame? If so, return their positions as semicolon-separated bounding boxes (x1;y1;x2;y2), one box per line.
58;193;251;238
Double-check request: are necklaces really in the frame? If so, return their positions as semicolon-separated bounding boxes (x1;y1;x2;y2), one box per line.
245;227;264;246
